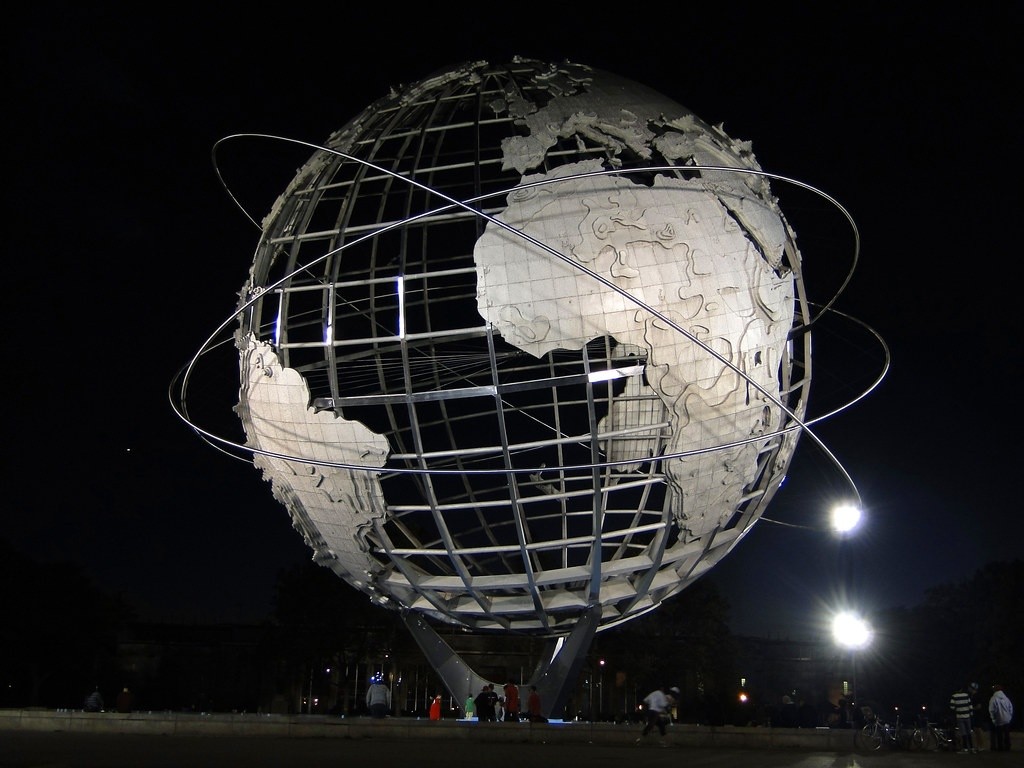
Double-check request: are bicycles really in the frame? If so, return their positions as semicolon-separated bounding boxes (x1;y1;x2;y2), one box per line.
860;712;975;752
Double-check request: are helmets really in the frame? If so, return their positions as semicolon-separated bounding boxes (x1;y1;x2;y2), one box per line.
372;675;384;684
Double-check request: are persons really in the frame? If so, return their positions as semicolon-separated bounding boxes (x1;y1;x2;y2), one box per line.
86;689;103;712
117;685;136;713
950;683;1012;753
366;680;390;718
430;695;441;720
465;679;542;722
640;683;679;736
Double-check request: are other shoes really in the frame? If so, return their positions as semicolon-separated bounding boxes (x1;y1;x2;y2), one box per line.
956;748;969;754
972;748;980;754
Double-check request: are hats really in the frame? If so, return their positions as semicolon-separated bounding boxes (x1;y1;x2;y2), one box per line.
481;685;490;691
783;696;791;702
489;683;494;689
